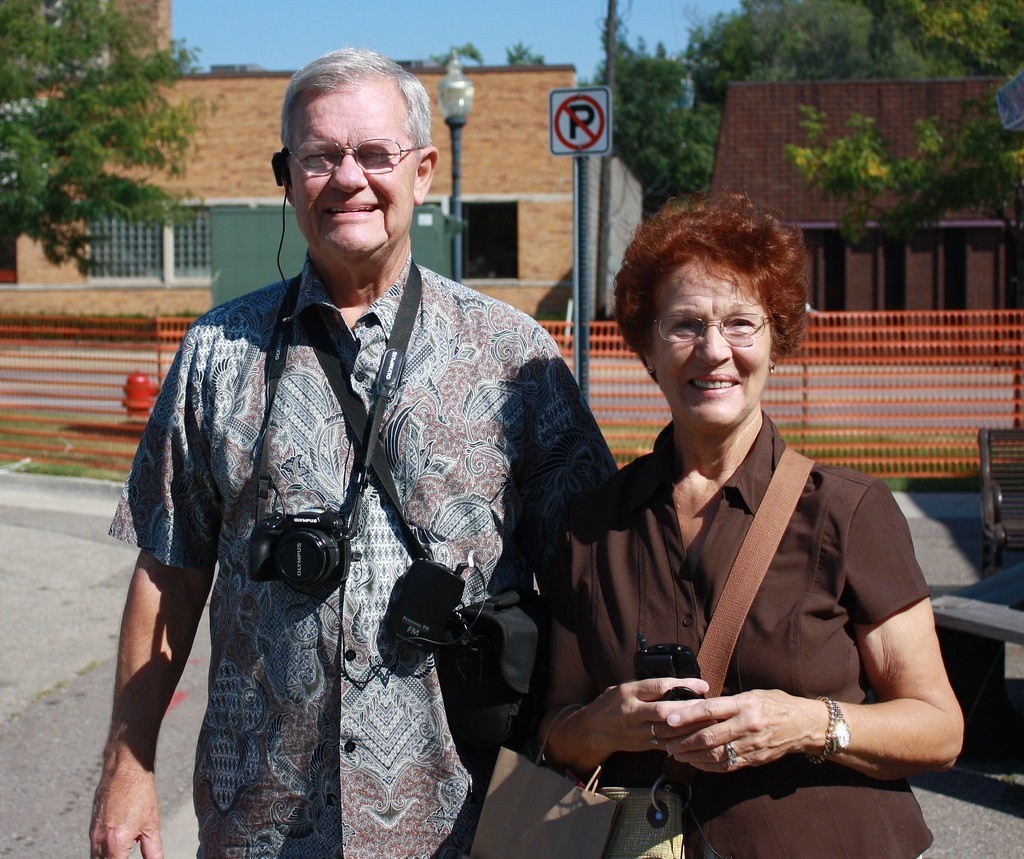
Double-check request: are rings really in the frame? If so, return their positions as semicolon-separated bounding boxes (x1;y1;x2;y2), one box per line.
648;724;660;745
725;742;737;766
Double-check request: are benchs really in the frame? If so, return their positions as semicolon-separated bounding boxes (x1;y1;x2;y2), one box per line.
977;424;1024;575
934;594;1024;708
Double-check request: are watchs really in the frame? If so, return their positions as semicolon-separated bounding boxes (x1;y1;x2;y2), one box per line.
807;695;851;765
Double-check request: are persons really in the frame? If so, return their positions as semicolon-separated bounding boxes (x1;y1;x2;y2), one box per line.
540;190;965;858
87;49;617;858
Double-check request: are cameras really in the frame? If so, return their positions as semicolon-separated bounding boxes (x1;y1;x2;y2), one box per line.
248;506;348;587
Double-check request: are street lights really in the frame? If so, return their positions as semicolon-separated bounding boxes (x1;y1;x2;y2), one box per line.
439;49;475;283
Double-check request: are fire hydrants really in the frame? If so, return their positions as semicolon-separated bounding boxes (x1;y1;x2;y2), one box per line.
122;370;160;422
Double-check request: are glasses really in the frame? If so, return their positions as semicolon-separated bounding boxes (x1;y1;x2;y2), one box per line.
651;314;772;343
285;139;425;174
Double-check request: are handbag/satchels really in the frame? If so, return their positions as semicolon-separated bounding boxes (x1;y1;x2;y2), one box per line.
442;589;549;759
469;704;619;859
600;785;687;859
